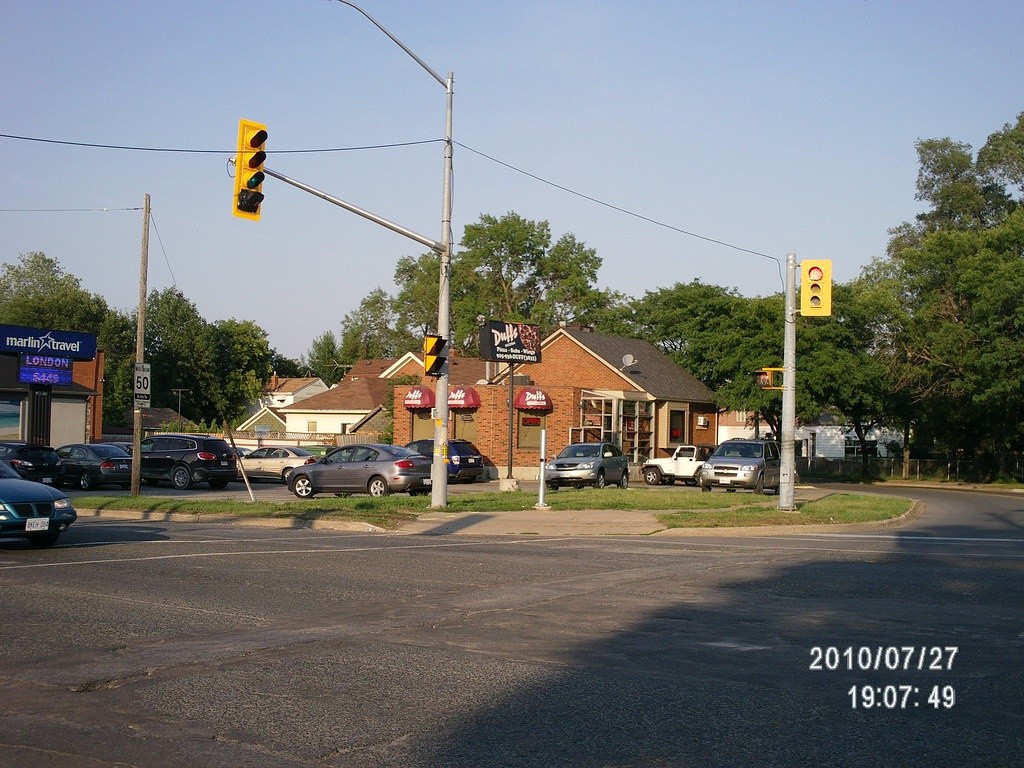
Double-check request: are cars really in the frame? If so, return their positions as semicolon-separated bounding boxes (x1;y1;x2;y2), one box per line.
236;445;320;485
229;446;261;478
0;460;77;545
102;442;149;456
53;443;142;491
286;444;434;499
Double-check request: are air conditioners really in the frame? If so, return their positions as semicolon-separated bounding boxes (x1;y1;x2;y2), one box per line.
697;416;708;426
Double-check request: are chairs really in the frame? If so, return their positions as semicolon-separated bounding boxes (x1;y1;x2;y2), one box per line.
754;448;761;458
726;448;742;456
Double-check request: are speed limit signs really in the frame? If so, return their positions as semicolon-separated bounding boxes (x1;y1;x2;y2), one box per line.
134;362;152;409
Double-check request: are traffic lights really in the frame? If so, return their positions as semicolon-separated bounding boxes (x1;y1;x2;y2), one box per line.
423;335;448;376
756;370;773;387
234;120;270;220
801;260;833;317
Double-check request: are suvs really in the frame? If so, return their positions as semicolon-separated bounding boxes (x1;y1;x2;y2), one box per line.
0;442;66;490
545;440;629;491
403;438;484;483
699;440;781;495
125;433;238;490
641;445;711;487
